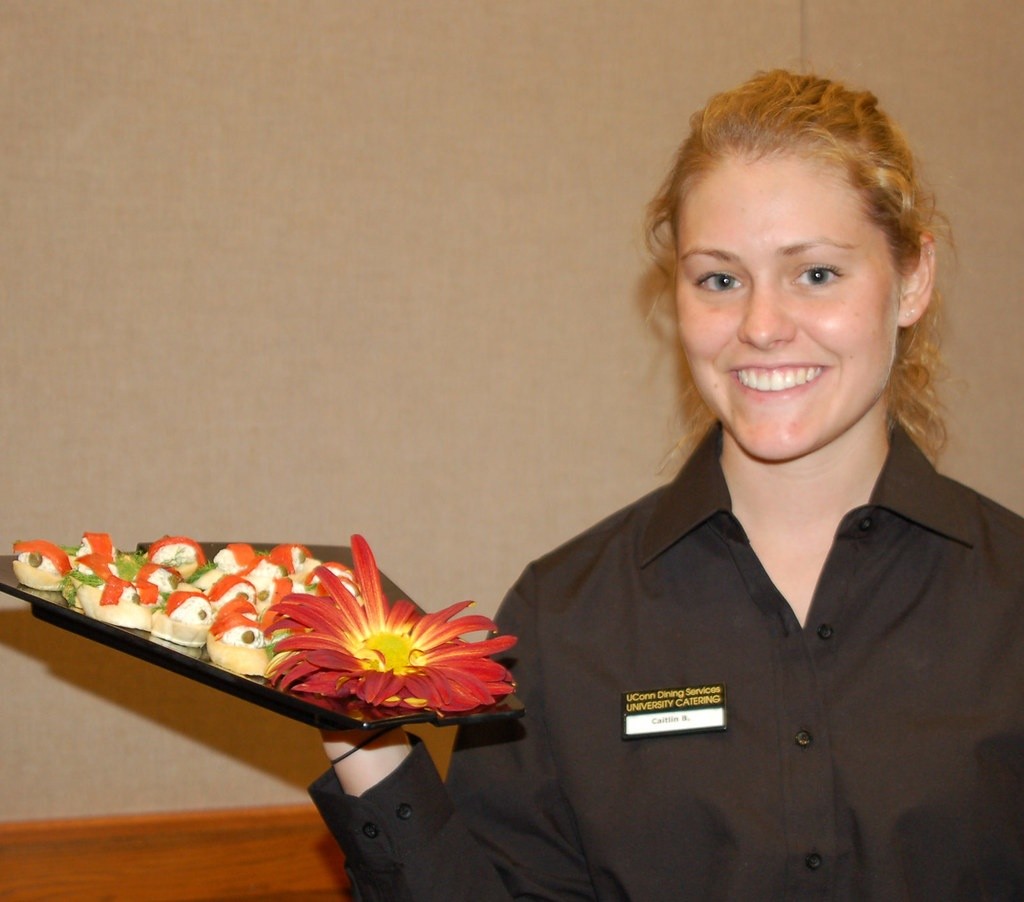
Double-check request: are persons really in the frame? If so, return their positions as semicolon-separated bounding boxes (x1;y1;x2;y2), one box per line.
308;67;1024;902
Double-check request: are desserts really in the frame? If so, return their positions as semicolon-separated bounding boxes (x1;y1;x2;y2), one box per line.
9;534;365;677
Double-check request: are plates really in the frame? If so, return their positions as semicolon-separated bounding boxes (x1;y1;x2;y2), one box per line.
0;541;527;722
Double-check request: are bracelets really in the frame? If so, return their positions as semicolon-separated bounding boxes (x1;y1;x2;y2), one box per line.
331;722;403;765
